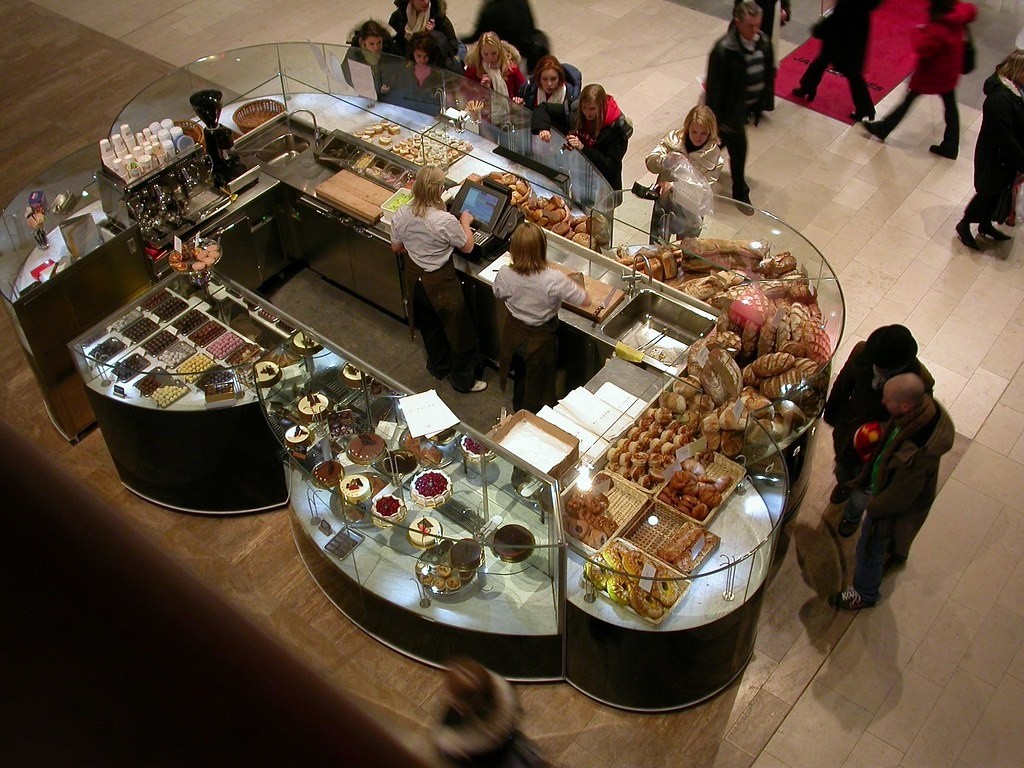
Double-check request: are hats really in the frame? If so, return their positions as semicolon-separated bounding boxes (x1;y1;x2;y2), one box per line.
858;323;918;369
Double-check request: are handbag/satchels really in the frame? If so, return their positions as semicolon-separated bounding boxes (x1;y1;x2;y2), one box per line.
959;39;976;74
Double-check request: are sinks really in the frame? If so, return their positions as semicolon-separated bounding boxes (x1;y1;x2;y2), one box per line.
254;133;310;167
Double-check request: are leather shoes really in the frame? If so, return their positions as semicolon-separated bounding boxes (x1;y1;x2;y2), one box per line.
732;195;755;215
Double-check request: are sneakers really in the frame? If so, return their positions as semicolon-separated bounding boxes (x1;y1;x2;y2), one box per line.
838;514;861;537
830;484;852;504
827;585;877;611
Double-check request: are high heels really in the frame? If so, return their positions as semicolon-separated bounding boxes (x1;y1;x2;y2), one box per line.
849;103;876;122
791;85;817;102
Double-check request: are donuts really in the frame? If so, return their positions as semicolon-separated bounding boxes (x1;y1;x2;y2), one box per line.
563;473;677;618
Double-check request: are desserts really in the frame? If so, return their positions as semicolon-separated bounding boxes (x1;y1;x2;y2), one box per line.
354;120;471;166
89;289;263;407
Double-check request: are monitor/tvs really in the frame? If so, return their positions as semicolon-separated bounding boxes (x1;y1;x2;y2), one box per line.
452;178;508;232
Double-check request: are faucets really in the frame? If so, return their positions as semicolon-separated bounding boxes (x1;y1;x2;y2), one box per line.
286;108;324;147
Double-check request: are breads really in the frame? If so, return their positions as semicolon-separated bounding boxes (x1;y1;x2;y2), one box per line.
481;172;610;252
618;237;831;457
607;416;733;572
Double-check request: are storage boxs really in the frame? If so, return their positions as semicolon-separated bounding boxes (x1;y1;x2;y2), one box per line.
488;409;579;477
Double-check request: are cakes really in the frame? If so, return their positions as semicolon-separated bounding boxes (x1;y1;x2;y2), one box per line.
256;361;282;388
342;363;372;388
293;331;323;354
284;394;533;590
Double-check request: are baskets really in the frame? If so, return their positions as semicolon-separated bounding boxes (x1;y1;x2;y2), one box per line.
171;118;206;155
604;436;697;500
652;451;747;529
579;537;693;627
563;469;650;556
621;500;721;582
231;98;286;134
484;170;532;206
520;195;570;231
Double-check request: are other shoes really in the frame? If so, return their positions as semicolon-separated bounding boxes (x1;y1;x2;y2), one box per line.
977;221;1011;241
928;144;959;160
956;222;980;251
470;380;487;392
862;120;891;141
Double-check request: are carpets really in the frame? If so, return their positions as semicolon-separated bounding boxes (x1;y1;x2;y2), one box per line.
774;0;929;126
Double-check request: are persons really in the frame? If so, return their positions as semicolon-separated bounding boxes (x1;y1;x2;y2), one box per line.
956;49;1024;250
792;0;883;122
340;16;406;102
388;0;455;58
823;324;935;538
492;222;592;415
390;166;488;393
705;0;791;216
408;31;464;75
827;373;955;611
531;84;628;208
428;658;552;768
513;55;574;137
861;0;978;160
463;31;526;131
645;105;726;245
